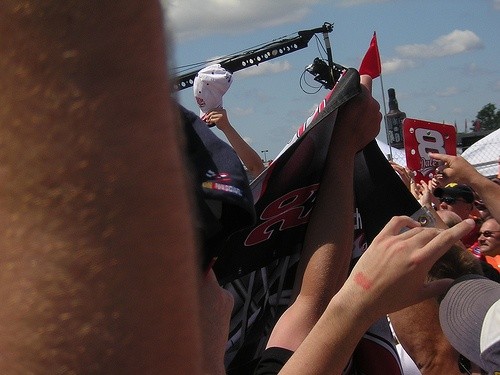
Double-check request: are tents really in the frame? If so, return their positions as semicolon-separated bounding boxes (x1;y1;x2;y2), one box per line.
460;127;500;178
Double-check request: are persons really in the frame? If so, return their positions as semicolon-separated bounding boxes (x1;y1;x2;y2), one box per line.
0;0;500;375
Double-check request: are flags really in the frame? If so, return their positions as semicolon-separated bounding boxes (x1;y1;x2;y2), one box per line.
358;33;381;78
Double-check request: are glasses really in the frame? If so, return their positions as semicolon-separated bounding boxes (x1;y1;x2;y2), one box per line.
457;351;474;375
438;196;467;205
476;230;500;238
477;205;487;210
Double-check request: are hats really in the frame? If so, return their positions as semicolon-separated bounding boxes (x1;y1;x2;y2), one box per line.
439;274;500;375
433;185;475;202
474;199;484;205
193;64;234;128
